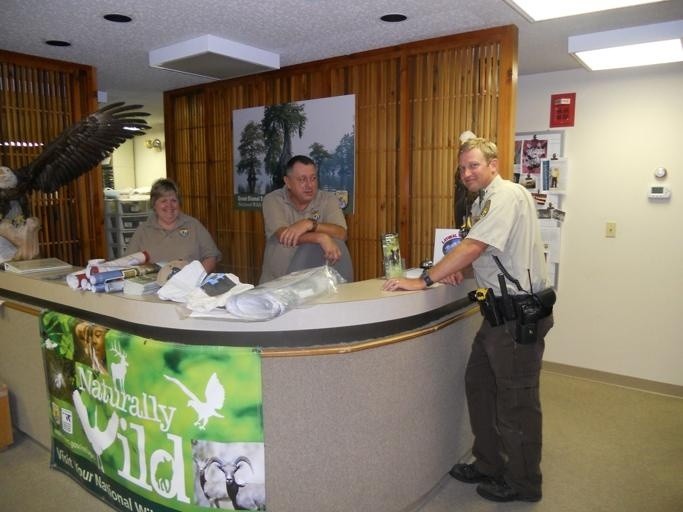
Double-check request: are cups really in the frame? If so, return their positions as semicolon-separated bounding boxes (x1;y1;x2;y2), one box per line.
86;258;106;280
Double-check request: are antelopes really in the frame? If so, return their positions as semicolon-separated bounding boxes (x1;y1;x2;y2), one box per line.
193;453;266;512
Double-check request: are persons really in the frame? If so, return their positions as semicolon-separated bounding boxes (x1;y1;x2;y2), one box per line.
262;155;348;266
125;179;222;275
383;137;556;502
91;326;105;366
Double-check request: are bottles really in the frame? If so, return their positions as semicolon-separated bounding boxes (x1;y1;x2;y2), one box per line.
380;232;403;283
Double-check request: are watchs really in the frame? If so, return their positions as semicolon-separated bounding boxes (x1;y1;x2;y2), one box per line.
421;269;433;287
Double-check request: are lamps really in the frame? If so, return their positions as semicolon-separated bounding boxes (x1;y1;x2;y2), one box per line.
503;0;681;72
148;34;280;80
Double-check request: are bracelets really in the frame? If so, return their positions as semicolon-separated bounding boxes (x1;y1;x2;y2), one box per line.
308;218;318;233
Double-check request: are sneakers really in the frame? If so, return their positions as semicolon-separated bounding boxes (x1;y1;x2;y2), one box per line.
478;477;542;503
450;458;488;482
